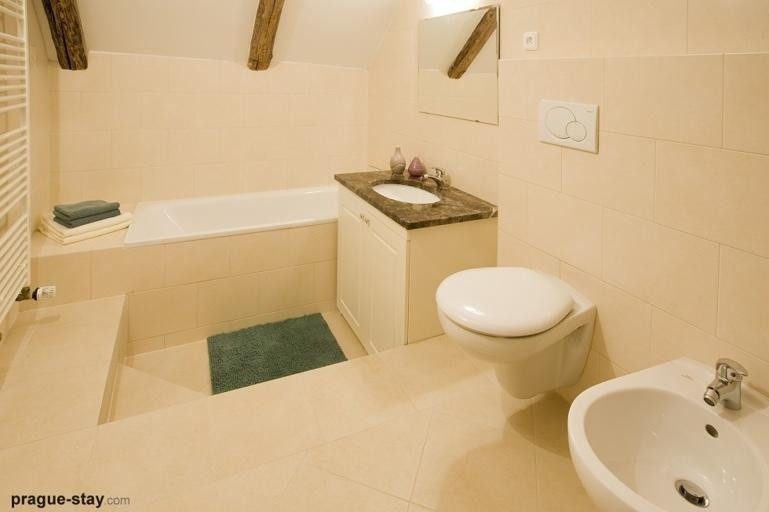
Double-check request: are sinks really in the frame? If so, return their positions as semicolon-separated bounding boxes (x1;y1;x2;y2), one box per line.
370;178;441;204
568;359;768;512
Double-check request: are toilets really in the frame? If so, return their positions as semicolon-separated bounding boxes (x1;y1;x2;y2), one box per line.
436;266;597;398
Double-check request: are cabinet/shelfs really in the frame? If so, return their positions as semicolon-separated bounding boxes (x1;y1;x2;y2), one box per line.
336;183;497;359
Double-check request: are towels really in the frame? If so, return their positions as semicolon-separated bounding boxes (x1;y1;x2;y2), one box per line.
38;198;133;246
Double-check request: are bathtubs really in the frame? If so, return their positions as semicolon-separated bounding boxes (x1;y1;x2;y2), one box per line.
123;185;340;245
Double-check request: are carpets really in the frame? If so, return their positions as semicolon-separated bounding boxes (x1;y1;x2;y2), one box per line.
207;310;348;394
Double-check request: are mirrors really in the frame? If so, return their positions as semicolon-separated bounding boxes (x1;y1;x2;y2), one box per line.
417;4;502;127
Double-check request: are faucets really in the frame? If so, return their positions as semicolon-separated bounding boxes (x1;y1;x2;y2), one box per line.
703;359;748;411
420;165;447;193
